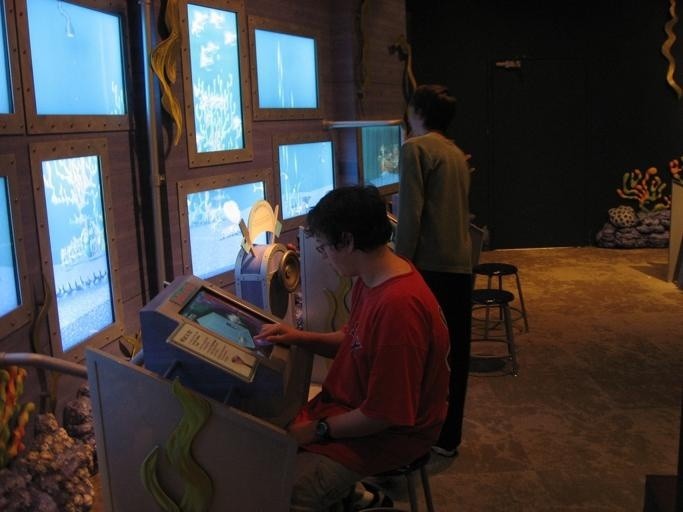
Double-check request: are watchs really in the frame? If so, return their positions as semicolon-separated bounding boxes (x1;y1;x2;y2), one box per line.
316;415;330;440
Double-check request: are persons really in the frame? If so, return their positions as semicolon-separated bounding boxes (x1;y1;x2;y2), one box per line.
251;185;453;511
390;86;474;455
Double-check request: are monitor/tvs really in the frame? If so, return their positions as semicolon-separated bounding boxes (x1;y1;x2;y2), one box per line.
178;284;278;361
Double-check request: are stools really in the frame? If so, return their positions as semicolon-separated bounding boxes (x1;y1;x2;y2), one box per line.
471;263;529;337
385;452;434;511
468;290;517;378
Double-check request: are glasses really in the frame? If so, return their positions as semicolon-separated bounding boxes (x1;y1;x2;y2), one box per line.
315;241;333;255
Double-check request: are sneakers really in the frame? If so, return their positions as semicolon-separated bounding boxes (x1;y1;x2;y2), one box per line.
431;444;454;457
351;491;393;512
